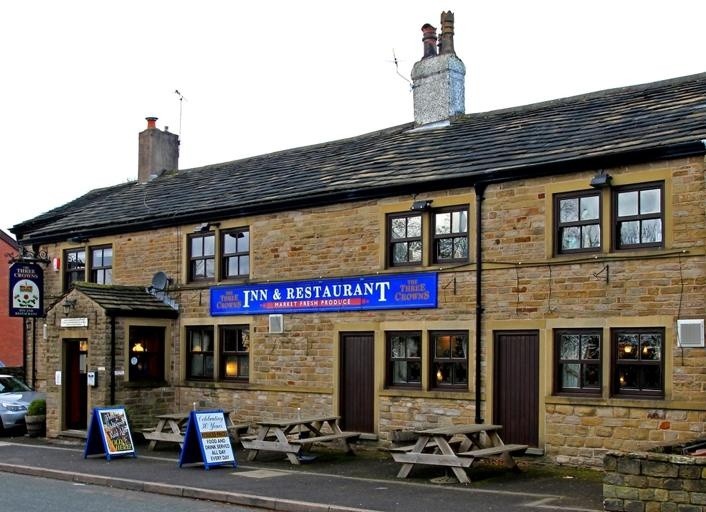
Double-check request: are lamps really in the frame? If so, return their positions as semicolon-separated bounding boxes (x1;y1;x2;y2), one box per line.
410;200;433;211
132;342;145;352
193;223;221;233
67;236;89;245
590;169;612;187
230;231;244;238
24;316;32;331
625;345;633;353
62;295;77;317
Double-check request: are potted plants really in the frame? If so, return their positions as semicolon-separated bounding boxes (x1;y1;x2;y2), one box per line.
25;399;46;437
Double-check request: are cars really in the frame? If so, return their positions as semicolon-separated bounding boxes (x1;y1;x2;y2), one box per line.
0;374;45;438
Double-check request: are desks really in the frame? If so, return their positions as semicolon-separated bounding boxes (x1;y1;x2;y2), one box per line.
396;424;520;484
241;416;354;465
142;410;239;451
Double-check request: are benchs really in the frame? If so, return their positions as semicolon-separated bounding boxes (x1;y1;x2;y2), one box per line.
456;444;529;457
227;424;249;429
288;433;361;444
140;426;187;433
240;431;310;441
389;441;462;461
